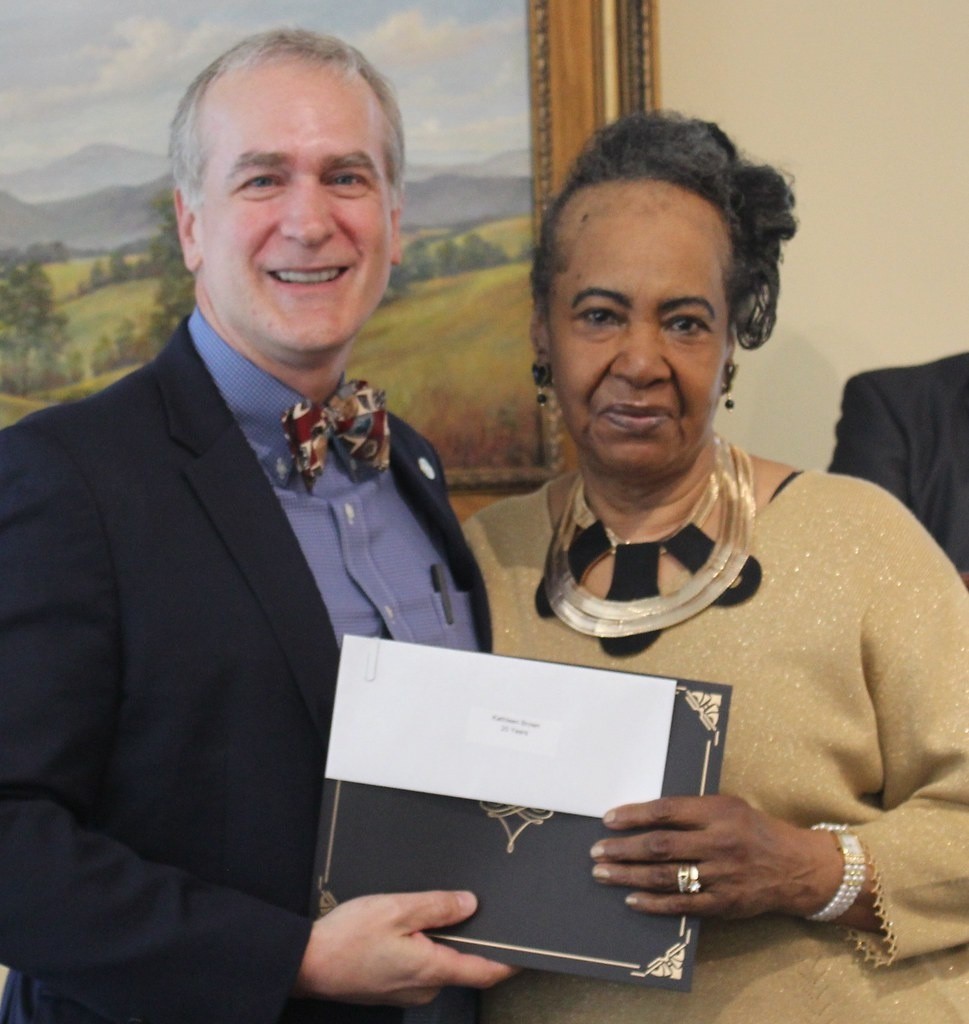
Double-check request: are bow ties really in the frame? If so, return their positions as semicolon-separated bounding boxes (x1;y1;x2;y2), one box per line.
283;383;389;492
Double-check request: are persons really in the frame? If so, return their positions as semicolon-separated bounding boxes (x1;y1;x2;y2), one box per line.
0;28;522;1024
460;113;968;1024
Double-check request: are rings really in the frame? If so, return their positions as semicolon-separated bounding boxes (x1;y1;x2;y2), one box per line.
689;864;701;893
678;864;689;893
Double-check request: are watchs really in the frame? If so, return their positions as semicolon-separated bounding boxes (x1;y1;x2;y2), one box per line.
806;822;866;921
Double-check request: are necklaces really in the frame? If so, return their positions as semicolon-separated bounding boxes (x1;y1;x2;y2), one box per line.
534;440;762;657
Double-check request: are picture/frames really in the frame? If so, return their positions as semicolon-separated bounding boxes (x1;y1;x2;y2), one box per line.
0;0;662;526
